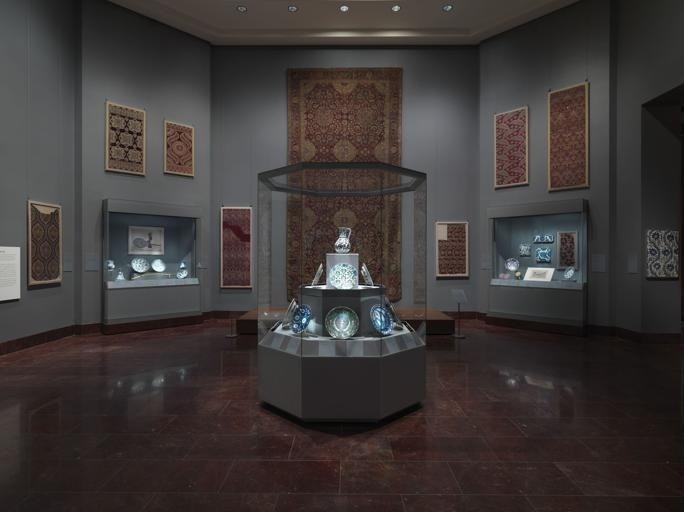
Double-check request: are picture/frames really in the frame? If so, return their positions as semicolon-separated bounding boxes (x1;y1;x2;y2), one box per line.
435;220;469;280
493;81;588;192
220;206;252;289
27;199;63;288
104;99;196;178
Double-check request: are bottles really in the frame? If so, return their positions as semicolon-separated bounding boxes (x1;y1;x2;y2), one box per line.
333;226;353;254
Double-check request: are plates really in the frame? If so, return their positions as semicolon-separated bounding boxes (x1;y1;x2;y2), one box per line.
152;258;166;273
329;263;359;292
309;262;324;286
281;298;312;336
130;257;150;273
367;297;405;335
362;263;375;286
504;258;521;274
562;265;577;281
325;306;360;341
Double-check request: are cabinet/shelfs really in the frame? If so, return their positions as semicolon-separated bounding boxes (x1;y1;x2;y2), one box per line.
101;198;202;336
486;198;589;337
254;160;429;429
